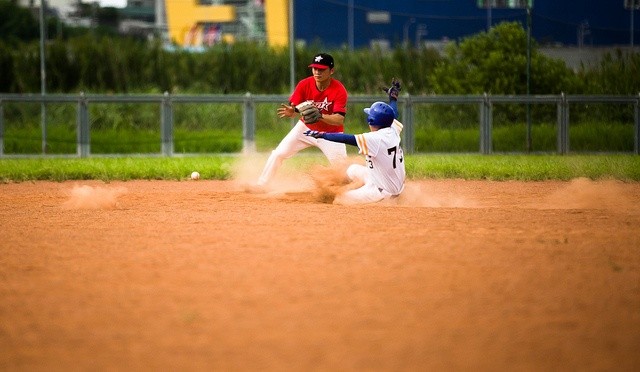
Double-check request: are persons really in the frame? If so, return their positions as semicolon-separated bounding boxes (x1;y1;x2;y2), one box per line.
259;53;348;187
304;78;406;206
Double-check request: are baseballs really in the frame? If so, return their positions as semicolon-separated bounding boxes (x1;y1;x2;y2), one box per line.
191;172;200;180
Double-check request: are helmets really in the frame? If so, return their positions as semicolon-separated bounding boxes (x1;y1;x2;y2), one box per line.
364;102;394;127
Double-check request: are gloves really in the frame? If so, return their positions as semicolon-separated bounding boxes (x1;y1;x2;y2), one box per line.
303;130;324;139
381;76;402;100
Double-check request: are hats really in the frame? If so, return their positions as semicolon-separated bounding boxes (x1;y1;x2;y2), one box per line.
307;53;334;69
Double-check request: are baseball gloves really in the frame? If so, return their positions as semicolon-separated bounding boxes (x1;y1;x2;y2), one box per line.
293;100;322;124
